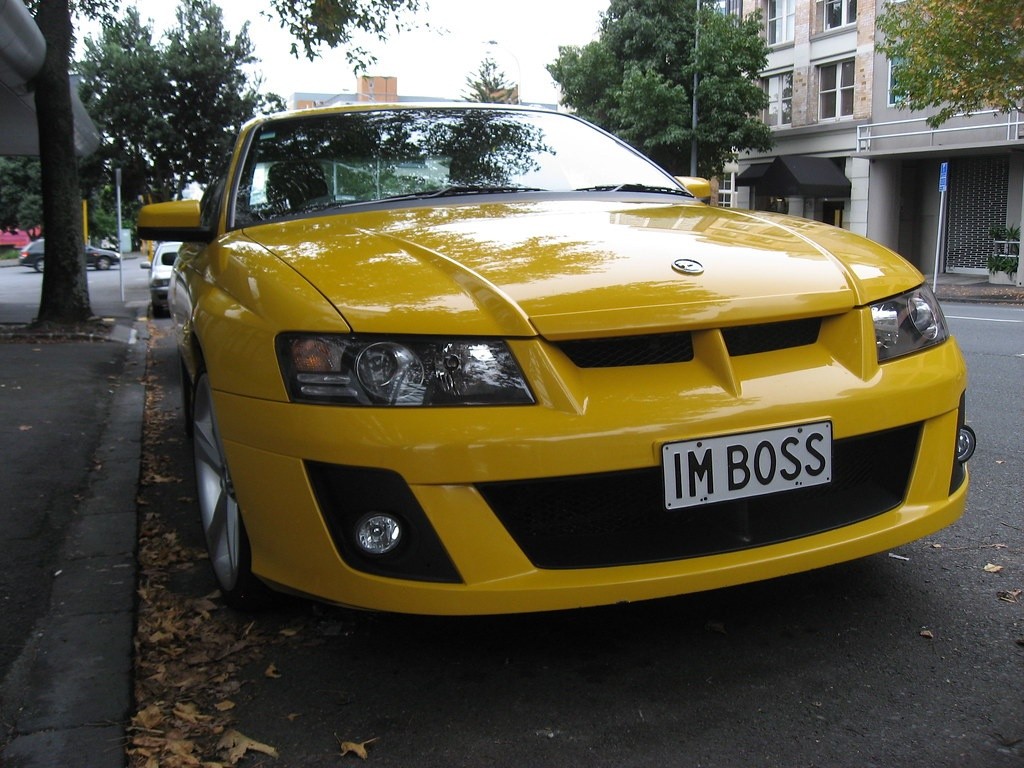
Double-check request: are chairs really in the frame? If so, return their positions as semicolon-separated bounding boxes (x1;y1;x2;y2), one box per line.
265;161;328;218
453;151;508;191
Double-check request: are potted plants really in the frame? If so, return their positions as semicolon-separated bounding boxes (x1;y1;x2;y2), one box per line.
986;223;1021;286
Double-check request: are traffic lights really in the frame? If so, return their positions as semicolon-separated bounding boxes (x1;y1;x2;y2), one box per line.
81;185;95;199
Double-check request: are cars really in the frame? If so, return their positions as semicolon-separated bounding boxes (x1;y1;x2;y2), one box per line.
19;237;119;272
133;99;976;621
140;241;185;317
102;238;119;248
